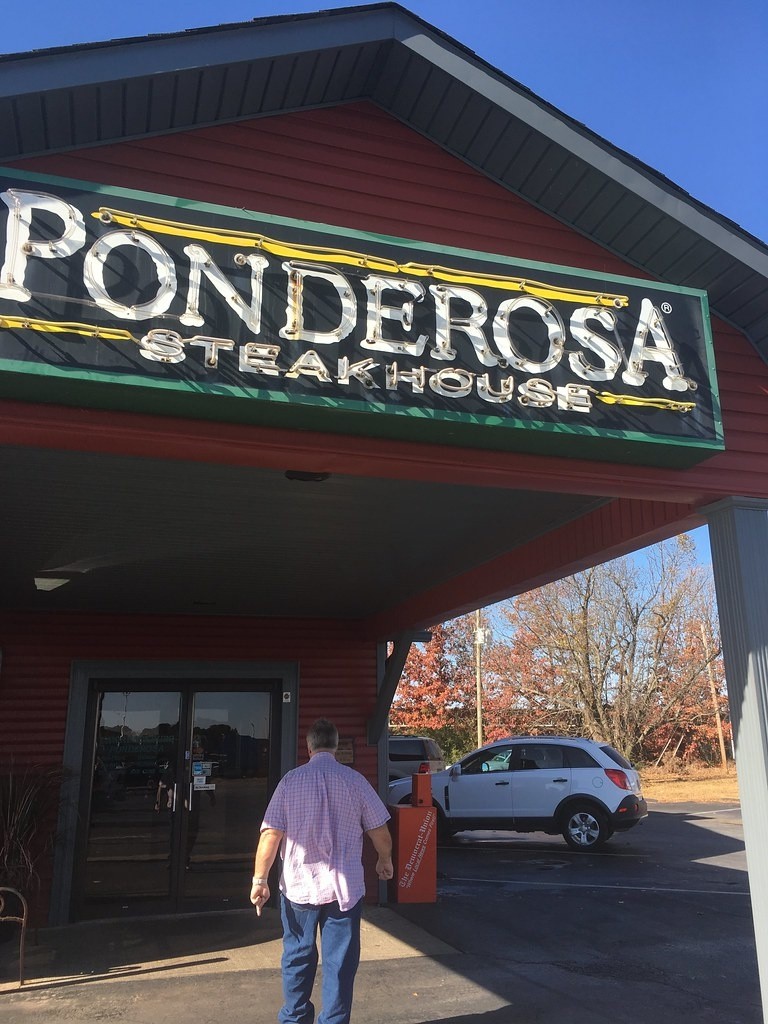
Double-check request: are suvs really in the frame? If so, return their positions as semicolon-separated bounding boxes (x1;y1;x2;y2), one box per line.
387;736;649;851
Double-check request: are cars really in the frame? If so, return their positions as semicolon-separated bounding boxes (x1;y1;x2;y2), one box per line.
482;747;563;772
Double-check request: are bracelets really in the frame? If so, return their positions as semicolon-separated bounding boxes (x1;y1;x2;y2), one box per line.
253;877;267;886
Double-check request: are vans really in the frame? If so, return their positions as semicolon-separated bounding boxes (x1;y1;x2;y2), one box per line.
386;737;446;781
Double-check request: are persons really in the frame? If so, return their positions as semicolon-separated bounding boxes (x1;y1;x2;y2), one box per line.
155;745;216;872
250;720;393;1024
506;755;523;769
522;759;537;768
